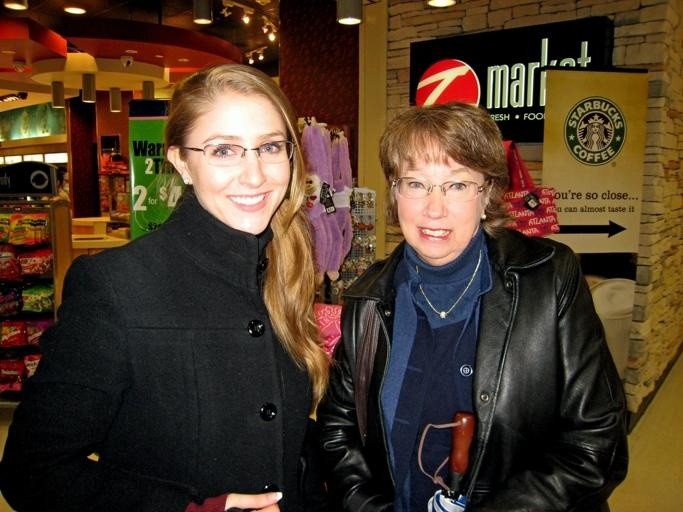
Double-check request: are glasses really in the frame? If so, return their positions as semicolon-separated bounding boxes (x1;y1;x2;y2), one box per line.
391;176;491;203
182;138;296;164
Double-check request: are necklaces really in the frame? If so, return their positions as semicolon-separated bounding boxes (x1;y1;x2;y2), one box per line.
412;251;482;323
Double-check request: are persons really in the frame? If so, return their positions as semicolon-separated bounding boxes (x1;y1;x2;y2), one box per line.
313;99;630;512
0;61;335;512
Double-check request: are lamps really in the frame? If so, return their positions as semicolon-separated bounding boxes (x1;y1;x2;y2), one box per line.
337;0;364;26
50;74;157;113
191;0;214;25
